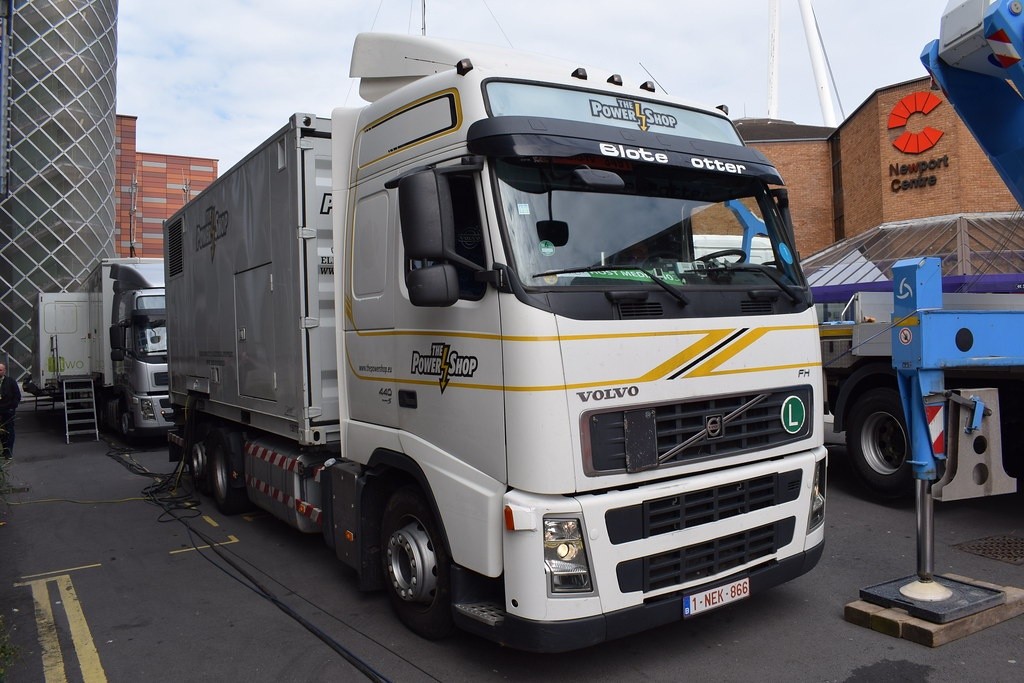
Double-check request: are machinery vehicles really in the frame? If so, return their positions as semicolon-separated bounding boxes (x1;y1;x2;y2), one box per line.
691;1;1023;626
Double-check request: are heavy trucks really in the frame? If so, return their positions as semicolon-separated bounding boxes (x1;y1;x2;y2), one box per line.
161;27;830;651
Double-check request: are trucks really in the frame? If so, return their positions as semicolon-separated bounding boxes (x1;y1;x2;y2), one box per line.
89;257;177;439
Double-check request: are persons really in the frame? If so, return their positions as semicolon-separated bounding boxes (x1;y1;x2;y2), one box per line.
0;364;21;459
